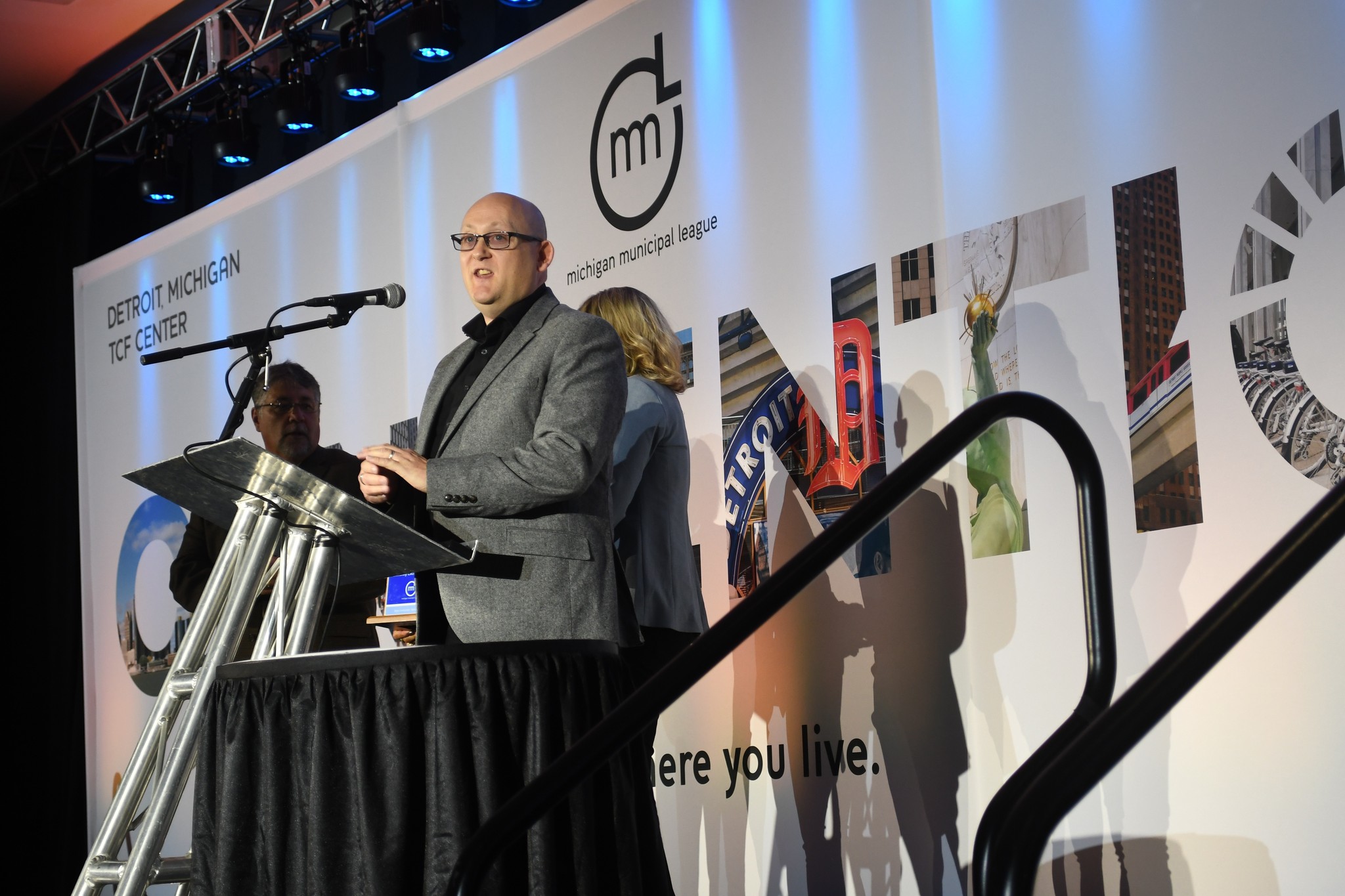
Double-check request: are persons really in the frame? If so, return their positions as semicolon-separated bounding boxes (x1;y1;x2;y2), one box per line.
361;194;629;654
578;285;711;643
169;360;371;654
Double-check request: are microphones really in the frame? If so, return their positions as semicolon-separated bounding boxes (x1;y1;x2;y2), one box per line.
304;281;407;319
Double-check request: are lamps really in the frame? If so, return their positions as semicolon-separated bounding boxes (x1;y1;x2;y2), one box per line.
137;0;543;203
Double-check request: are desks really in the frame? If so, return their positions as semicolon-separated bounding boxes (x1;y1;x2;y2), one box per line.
185;638;674;896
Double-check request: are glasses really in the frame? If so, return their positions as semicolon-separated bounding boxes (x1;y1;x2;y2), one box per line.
255;398;322;413
450;231;541;251
